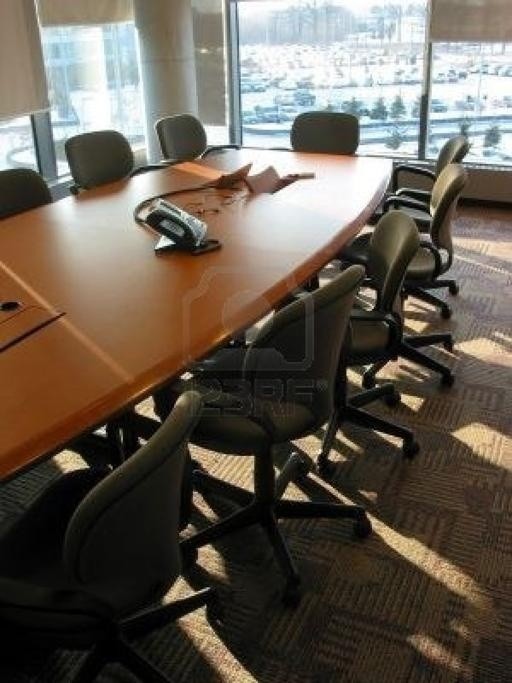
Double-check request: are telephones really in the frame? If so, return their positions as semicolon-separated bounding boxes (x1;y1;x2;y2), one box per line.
145;197;208;251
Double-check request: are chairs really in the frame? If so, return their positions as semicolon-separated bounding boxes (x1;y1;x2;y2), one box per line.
64;129;170;196
155;114;240;164
340;163;470;389
1;169;53;220
153;264;372;611
273;211;421;477
1;390;227;682
289;113;360;155
366;134;470;318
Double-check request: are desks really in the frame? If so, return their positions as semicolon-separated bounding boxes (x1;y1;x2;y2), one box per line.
2;148;394;481
